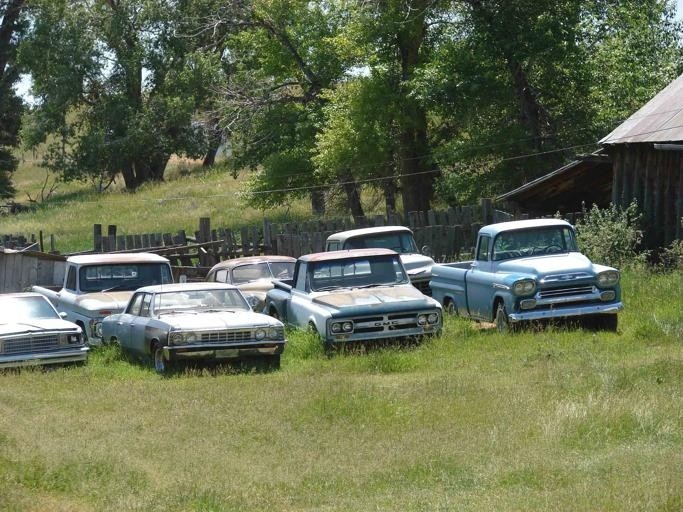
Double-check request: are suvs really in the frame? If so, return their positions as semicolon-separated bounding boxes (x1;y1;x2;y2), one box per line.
325;225;434;298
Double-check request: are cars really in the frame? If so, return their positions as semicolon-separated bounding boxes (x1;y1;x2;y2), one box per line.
203;254;301;316
0;290;91;372
101;282;286;377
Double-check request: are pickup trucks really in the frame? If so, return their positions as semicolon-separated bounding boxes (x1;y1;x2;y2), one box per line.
428;216;624;336
263;248;444;357
30;252;188;350
